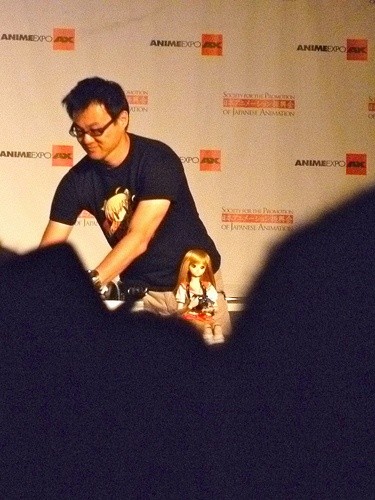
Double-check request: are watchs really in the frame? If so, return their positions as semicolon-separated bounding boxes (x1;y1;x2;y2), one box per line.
87;267;101;291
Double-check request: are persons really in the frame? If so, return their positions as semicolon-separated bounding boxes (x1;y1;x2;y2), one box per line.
176;251;225;344
38;77;233;337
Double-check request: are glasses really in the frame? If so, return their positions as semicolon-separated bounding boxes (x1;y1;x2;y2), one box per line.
69;113;119;138
